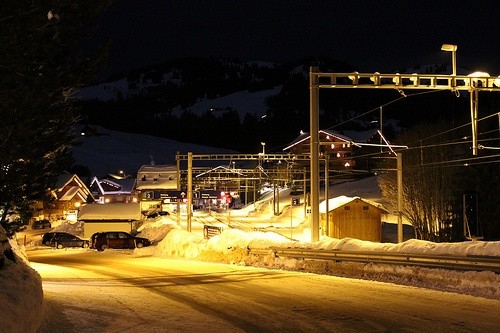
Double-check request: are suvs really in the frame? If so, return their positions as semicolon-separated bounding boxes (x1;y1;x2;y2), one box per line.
89;231;151;252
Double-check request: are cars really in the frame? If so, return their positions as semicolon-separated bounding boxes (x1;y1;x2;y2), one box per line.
32;219;52;230
42;230;89;250
